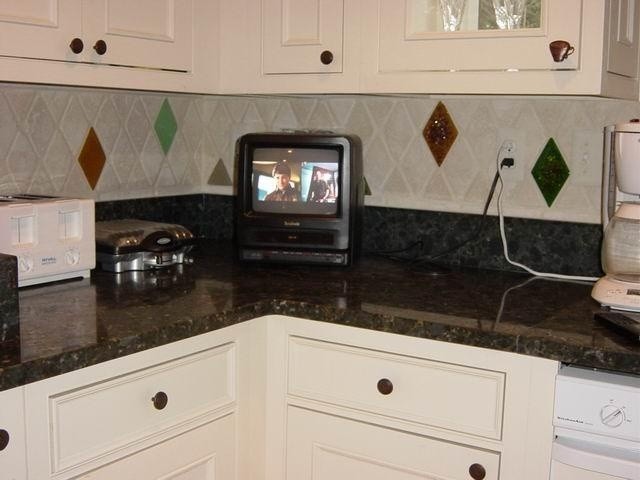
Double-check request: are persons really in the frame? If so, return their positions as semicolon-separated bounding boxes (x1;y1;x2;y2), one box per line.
310;170;330;203
264;163;302;203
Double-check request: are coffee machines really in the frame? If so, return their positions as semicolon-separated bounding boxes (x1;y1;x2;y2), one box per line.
588;116;640;314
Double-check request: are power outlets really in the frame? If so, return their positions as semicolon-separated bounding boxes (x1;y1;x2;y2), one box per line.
492;129;523;184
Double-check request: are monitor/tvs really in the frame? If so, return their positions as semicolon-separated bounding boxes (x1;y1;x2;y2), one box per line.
234;130;364;267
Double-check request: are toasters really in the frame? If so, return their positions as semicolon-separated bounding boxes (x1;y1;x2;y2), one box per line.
0;193;98;287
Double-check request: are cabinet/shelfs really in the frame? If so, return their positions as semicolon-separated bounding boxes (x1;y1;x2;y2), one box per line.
0;314;269;480
0;1;219;94
272;314;559;480
219;0;640;99
